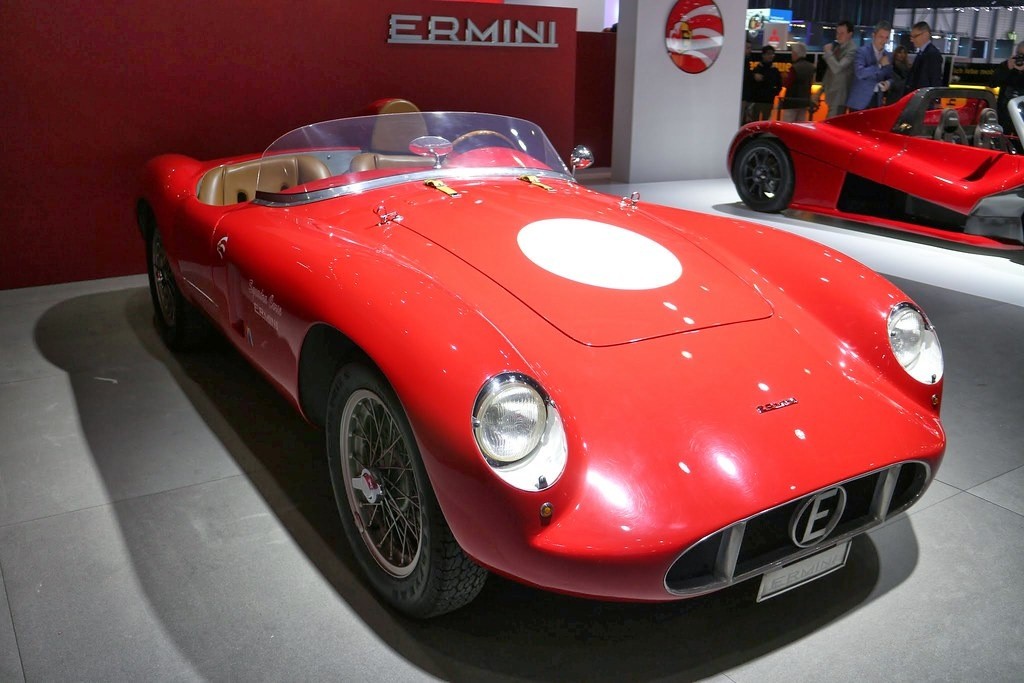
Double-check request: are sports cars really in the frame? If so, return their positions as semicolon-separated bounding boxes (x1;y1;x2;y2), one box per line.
727;81;1024;266
137;101;947;620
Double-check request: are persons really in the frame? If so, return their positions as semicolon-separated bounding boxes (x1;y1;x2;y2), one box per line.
886;45;912;107
905;21;944;93
845;20;895;113
739;41;760;123
989;39;1024;136
782;42;815;121
819;21;857;119
752;45;782;121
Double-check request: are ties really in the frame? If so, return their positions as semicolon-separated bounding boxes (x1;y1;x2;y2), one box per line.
910;50;921;71
831;47;840;55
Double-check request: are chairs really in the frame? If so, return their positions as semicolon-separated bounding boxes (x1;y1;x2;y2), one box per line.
974;108;1016;154
350;99;445;175
933;108;968;145
197;154;331;204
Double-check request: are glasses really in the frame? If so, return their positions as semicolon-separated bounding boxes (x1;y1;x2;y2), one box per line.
909;31;926;39
